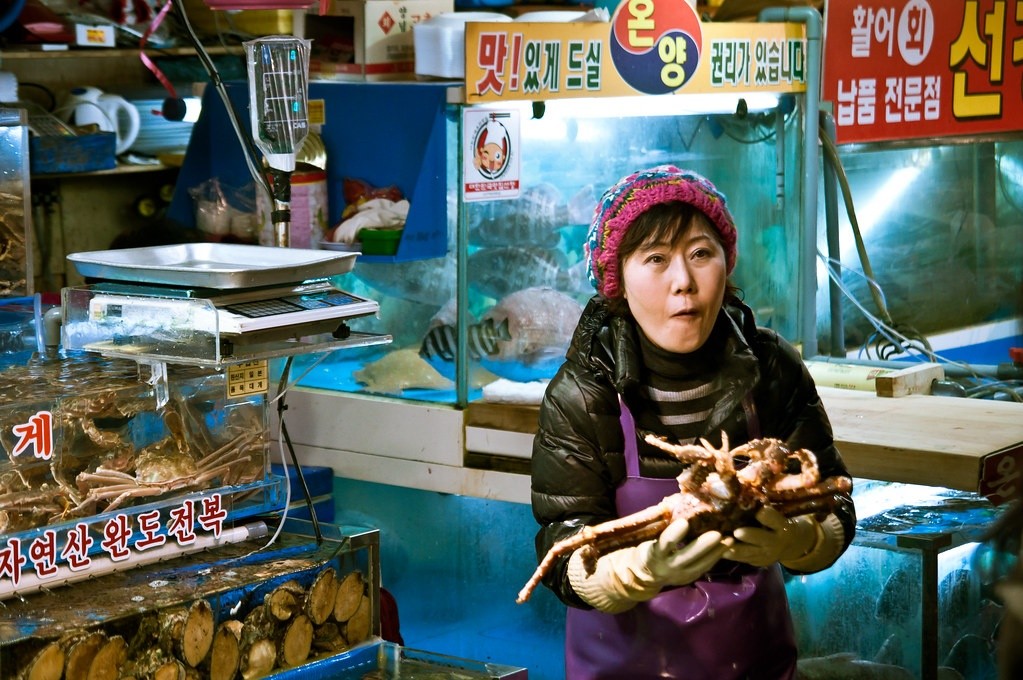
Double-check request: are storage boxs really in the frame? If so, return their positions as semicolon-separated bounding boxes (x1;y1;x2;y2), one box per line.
191;81;1022;469
0;516;382;680
0;108;37;298
816;383;1022;493
292;0;455;82
29;131;116;175
331;477;1023;680
0;376;289;582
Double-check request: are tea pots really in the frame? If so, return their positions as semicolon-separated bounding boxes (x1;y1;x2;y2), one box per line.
58;85;140;157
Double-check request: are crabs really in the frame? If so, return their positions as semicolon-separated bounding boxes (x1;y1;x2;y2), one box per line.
515;432;854;607
0;347;269;547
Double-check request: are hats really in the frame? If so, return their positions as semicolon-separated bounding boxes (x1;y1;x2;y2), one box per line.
584;164;736;299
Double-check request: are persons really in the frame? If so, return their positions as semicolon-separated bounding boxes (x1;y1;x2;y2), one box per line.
531;165;858;680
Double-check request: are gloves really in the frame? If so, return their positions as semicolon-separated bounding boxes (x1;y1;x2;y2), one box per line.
567;518;735;615
721;505;844;574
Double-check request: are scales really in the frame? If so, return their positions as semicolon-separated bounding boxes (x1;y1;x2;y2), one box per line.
65;243;379;355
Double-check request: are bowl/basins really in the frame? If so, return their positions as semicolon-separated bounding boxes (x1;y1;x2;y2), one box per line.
211;5;308;54
358;228;404;255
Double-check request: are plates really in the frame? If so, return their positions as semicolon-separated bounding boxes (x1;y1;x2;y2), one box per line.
126;98;196;153
318;242;362;253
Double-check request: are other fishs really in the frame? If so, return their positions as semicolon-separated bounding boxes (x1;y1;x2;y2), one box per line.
350;173;587;401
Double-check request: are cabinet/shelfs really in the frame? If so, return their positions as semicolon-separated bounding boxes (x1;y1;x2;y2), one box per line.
0;45;243;179
